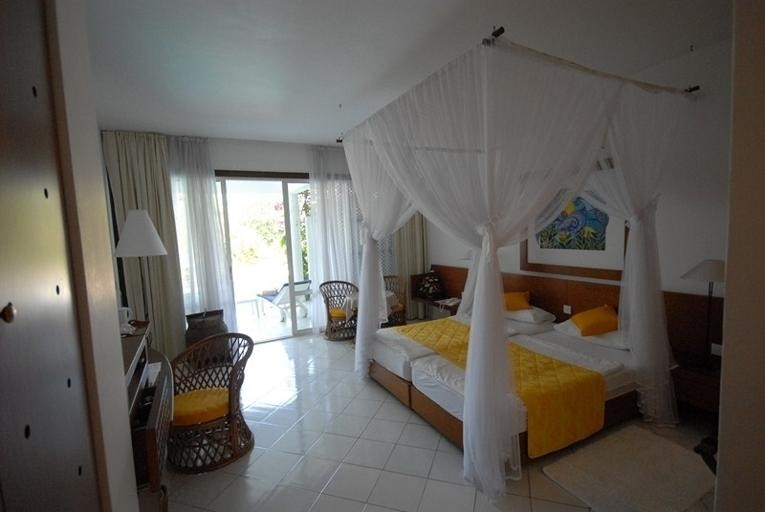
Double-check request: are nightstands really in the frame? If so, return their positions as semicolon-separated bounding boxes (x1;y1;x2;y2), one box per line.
670;360;721;455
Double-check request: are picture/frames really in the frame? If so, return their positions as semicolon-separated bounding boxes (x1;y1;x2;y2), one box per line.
518;158;630;281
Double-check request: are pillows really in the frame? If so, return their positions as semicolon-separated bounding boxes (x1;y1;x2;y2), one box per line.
553;316;630;351
500;290;534;312
568;302;621;337
497;303;557;325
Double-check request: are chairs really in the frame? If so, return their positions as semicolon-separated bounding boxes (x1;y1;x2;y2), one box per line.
157;329;257;478
317;280;358;343
256;279;313;324
381;273;409;327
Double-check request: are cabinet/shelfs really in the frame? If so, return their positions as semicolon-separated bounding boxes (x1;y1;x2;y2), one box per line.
120;319;176;511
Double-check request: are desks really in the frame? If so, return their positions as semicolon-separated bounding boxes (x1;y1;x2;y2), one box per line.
336;289;400;325
410;288;464;321
235;297;261;321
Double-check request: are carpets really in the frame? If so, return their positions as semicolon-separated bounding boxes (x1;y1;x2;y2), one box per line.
539;420;716;512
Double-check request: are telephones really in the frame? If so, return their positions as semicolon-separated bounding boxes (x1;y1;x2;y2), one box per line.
444;297;461;307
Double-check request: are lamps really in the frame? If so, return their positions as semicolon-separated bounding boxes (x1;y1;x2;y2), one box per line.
679;258;726;355
110;207;169;323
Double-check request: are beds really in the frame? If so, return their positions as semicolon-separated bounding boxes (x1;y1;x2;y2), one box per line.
367;315;559;410
409;328;640;470
